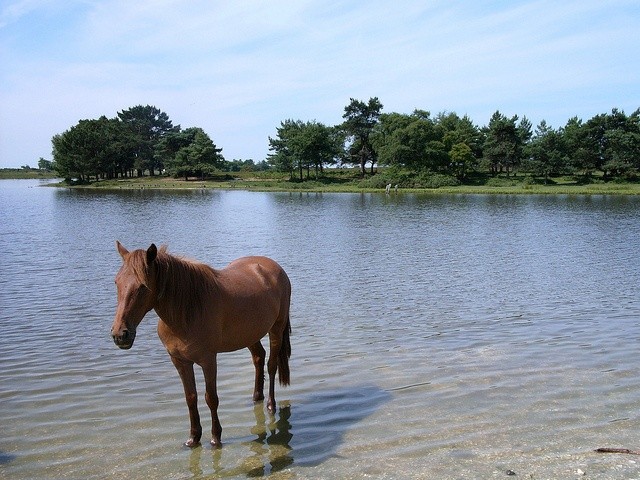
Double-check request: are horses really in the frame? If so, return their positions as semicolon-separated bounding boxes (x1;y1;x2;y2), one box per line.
111;240;292;450
